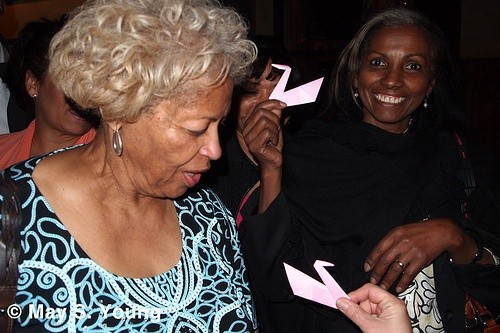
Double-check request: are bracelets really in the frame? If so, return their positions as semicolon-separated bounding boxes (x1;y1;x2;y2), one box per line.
448;228;484;267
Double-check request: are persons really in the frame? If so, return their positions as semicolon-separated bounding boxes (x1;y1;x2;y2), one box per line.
0;36;12;134
0;0;259;333
336;283;413;333
200;36;297;224
0;18;96;172
237;8;500;333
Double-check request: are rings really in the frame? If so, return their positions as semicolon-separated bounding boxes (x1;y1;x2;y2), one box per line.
395;259;407;270
